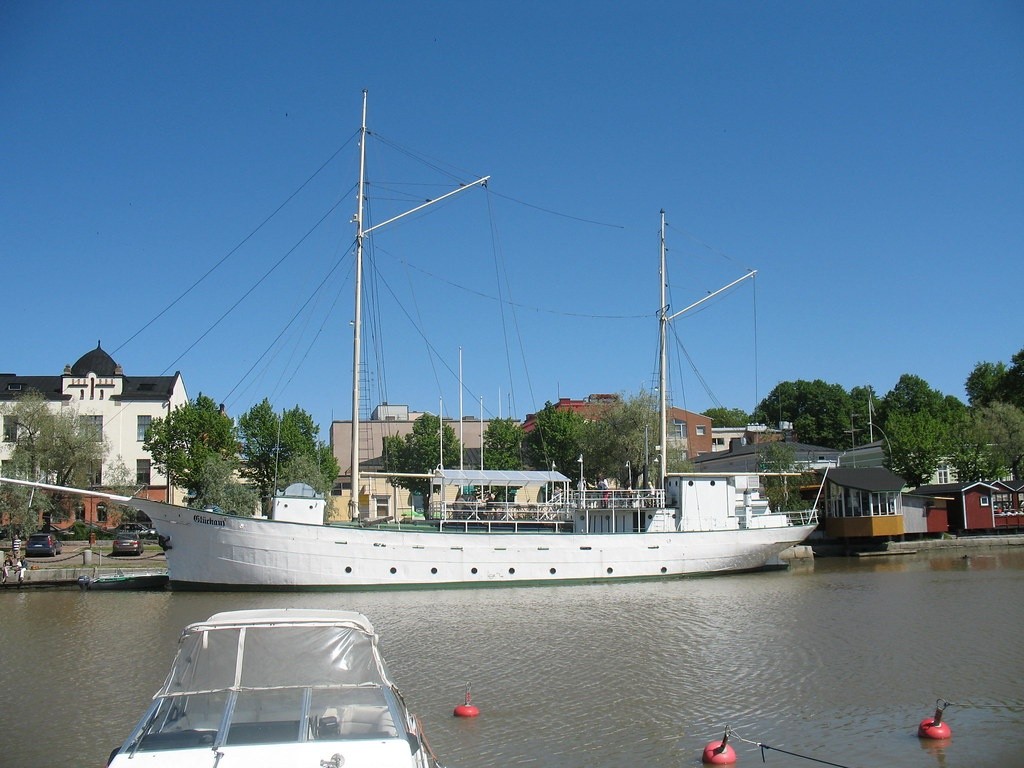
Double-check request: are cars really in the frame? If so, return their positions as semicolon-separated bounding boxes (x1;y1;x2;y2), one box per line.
106;521;158;540
26;533;63;557
110;533;144;555
0;523;76;539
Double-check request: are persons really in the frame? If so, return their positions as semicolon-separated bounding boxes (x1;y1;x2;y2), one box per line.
819;491;1024;516
2;534;28;584
454;475;655;522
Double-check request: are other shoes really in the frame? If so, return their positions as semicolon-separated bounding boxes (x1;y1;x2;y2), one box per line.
647;504;652;507
2;578;6;583
603;504;608;508
20;578;24;582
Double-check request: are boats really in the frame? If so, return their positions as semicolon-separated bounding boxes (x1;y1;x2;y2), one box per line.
103;607;441;767
2;88;832;590
